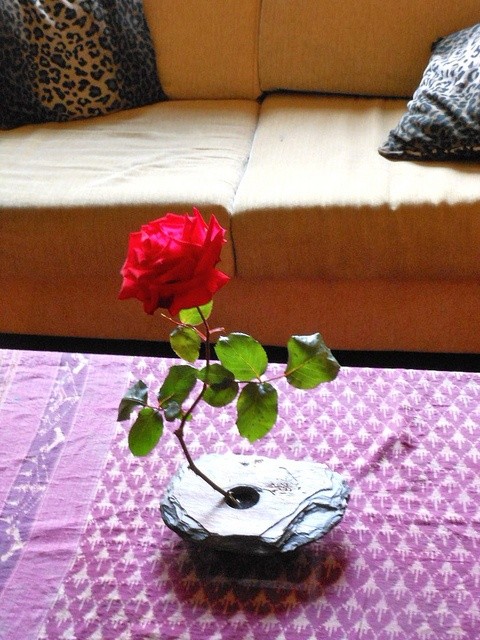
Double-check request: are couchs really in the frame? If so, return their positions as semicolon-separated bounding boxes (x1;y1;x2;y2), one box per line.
1;0;480;355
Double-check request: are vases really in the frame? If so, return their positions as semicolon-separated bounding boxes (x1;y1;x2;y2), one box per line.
158;452;345;556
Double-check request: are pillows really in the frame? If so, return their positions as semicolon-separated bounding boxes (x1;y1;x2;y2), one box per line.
377;20;480;162
0;0;168;132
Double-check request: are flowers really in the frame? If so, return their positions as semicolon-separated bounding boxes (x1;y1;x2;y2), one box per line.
115;207;343;507
